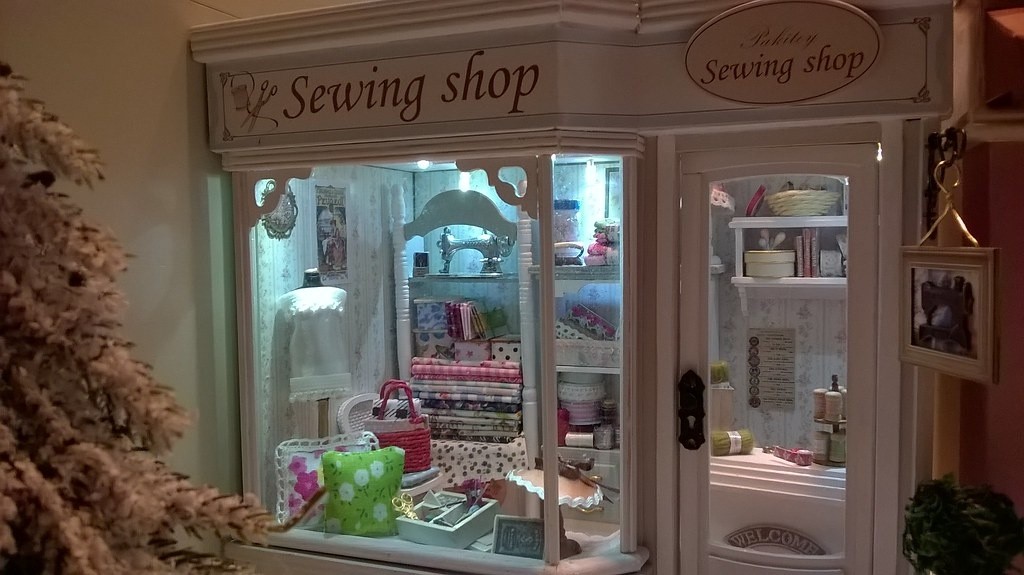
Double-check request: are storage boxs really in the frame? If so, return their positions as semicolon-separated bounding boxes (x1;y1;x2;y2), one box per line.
414;329;454;358
413;298;458;330
454;337;498;361
395;491;500;550
556;340;621;367
492;335;521;362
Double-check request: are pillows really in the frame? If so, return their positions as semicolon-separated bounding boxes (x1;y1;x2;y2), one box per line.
319;446;405;536
275;430;380;529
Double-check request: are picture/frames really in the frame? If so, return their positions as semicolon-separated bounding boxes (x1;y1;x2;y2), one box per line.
606;168;621;219
899;246;1000;386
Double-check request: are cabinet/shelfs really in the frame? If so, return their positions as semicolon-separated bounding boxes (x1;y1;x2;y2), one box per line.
187;0;987;575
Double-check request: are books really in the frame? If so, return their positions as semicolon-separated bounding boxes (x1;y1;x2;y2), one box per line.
796;228;819;276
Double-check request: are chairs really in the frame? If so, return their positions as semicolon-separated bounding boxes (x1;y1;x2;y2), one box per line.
337;393;444;504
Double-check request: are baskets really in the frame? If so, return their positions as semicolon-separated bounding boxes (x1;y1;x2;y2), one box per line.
763;190;842;217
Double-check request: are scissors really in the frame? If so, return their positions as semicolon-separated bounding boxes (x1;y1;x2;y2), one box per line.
392;493;419;522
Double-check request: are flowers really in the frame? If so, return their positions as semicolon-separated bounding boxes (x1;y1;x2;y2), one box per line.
0;61;277;575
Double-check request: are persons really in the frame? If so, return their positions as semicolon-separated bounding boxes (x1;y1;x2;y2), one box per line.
280;267;354;403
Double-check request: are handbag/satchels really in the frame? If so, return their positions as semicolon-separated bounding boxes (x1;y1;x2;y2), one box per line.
366;379;432;473
